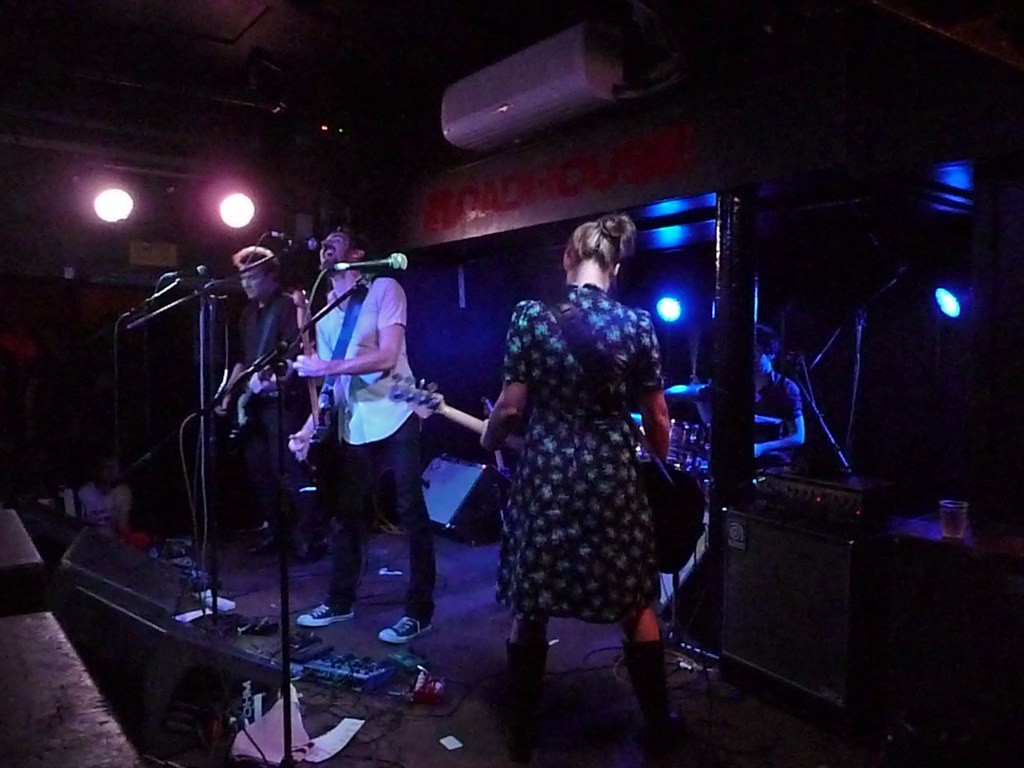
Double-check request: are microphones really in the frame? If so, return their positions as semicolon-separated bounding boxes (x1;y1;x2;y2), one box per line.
159;265;207;281
266;231;319;249
333;252;408;272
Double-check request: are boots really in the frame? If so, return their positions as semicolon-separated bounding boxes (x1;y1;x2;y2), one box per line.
506;636;553;730
622;630;685;752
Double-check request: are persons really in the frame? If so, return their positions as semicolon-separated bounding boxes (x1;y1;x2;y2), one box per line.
696;323;803;466
212;245;331;563
478;209;694;755
76;449;133;538
286;224;437;643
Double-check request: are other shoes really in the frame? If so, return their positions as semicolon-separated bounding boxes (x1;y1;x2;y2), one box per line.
247;523;279;556
291;519;317;556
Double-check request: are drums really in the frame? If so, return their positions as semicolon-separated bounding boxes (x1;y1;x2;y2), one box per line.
633;417;710;493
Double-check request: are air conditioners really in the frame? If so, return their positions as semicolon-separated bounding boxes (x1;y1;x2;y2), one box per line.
440;23;623;150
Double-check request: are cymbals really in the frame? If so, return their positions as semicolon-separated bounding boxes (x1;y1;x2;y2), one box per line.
665;383;762;403
754;415;782;427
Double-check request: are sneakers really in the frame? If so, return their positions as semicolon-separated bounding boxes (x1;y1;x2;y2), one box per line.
378;617;432;644
296;602;354;627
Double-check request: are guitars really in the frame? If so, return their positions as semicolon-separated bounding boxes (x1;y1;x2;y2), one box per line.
216;327;314;447
389;371;707;574
289;288;339;512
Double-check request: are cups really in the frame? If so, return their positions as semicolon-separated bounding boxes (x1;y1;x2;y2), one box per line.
940;500;968;537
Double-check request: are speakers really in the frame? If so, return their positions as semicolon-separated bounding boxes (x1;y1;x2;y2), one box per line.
722;496;927;707
420;454;511;543
